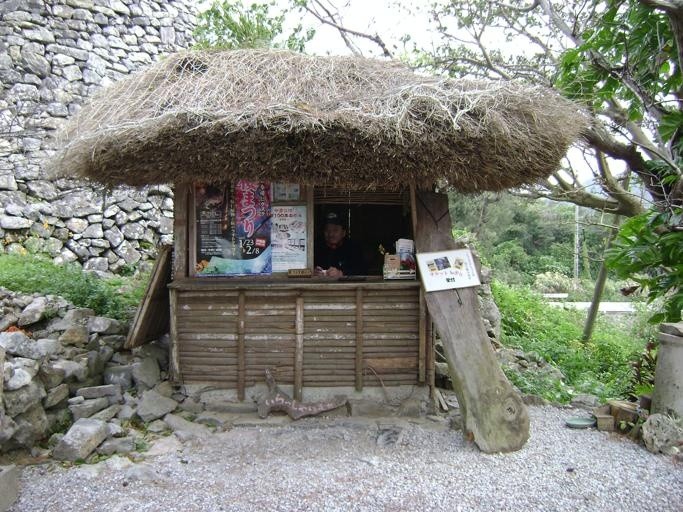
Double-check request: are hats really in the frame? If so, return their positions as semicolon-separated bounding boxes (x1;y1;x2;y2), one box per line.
323;212;341;223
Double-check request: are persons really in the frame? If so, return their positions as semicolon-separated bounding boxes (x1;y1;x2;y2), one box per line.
313;212;366;280
